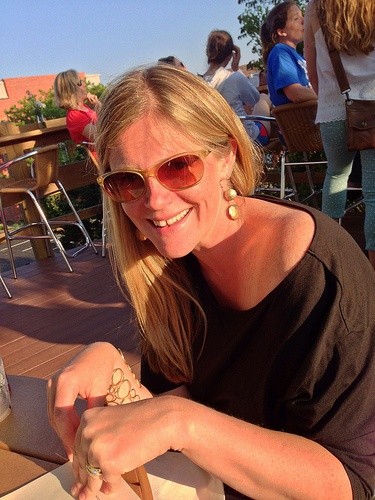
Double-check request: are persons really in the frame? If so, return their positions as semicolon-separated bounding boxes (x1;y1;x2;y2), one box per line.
303;0;375;267
259;2;318;108
42;62;375;500
160;56;186;75
203;30;275;146
54;69;104;167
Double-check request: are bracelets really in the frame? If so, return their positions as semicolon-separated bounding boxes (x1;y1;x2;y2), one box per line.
102;346;141;407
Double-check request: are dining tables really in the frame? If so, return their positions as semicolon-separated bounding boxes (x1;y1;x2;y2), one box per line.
0;375;226;499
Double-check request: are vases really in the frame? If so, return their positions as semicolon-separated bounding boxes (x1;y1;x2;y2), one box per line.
37;114;48;129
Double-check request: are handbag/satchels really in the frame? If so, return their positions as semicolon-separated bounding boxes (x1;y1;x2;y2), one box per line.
346;99;375;151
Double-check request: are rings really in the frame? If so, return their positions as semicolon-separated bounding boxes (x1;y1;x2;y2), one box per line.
84;463;102;479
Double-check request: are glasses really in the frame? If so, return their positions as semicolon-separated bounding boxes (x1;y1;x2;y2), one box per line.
77;80;82;86
177;62;184;68
97;143;218;203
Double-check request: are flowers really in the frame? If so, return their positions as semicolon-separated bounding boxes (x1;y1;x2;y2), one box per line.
30;101;46;121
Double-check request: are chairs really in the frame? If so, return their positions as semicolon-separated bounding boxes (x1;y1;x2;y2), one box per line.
270;99;366;226
0;144;98;278
236;114;298;204
